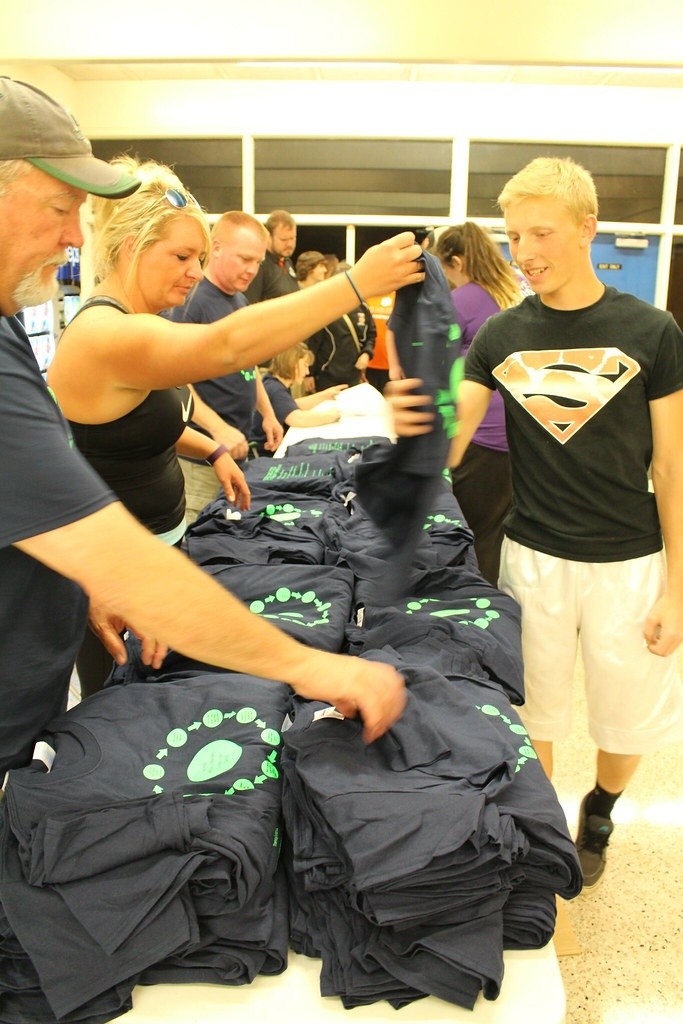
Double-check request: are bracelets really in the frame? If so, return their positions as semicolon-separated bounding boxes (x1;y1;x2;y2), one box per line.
204;445;229;467
343;270;364;304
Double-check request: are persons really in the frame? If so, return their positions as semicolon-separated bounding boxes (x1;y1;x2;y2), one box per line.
247;340;349;460
241;211;300;379
384;227;434;382
45;157;427;709
433;222;524;589
292;252;377;397
0;75;408;771
382;155;683;886
156;211;284;531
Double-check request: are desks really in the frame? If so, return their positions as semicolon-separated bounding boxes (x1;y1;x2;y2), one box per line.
110;397;570;1024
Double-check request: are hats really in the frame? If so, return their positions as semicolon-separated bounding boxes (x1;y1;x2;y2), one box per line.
0;75;142;199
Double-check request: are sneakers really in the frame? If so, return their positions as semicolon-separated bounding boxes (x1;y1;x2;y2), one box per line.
574;791;614;889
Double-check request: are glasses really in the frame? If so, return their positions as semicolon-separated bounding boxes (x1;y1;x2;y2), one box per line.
137;188;201;218
